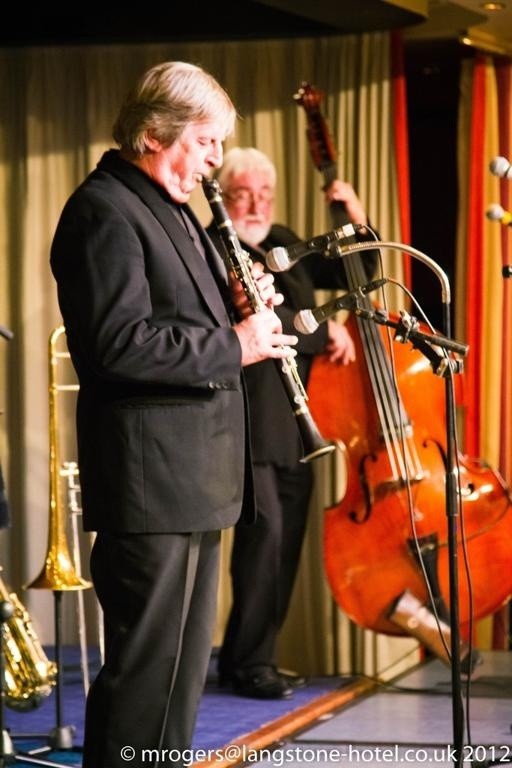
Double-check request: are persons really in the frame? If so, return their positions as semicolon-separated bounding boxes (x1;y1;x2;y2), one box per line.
204;146;382;701
47;59;300;768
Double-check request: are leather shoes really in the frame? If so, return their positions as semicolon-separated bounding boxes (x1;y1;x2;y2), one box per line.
220;665;307;699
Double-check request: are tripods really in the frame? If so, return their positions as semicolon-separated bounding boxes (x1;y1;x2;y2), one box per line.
7;591;90;757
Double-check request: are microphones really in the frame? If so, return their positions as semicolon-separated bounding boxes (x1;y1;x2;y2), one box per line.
490;157;511;174
294;281;388;333
485;203;510;228
264;221;359;274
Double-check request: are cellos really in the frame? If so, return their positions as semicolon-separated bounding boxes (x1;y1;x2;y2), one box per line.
293;79;512;637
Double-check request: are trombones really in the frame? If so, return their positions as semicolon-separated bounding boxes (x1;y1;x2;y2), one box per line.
23;318;112;696
